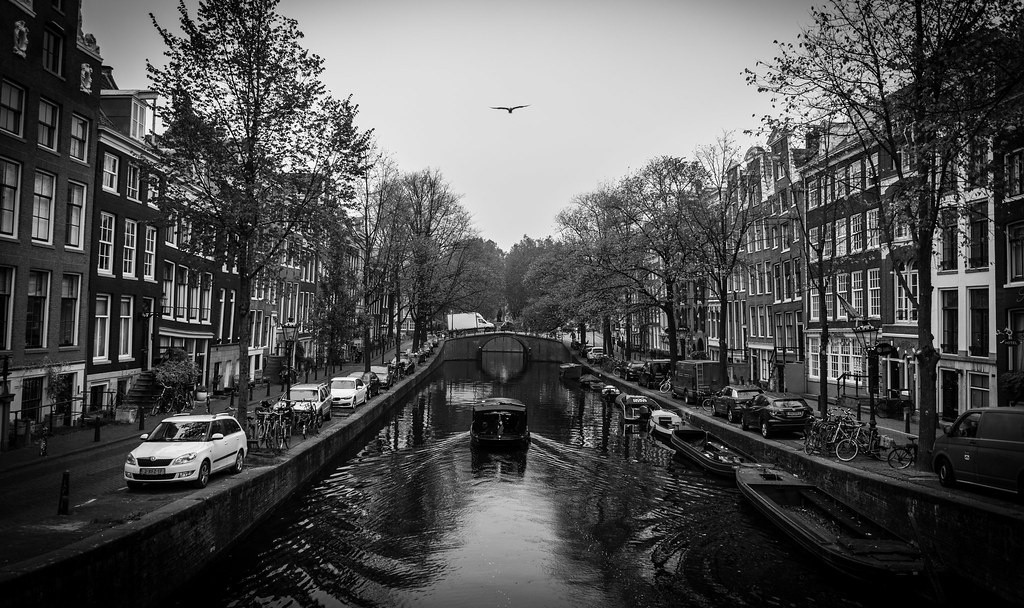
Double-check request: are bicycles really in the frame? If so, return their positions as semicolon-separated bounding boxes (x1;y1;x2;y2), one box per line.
702;392;718;412
146;381;327;454
875;388;915;420
660;370;672;393
596;355;629;376
802;408;918;470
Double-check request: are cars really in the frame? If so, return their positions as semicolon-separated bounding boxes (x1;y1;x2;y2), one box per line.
331;377;368;409
417;331;449;363
586;346;604;363
620;360;647;382
346;370;382;399
739;391;814;438
391;355;415;376
710;385;766;422
638;360;672;390
371;365;394;390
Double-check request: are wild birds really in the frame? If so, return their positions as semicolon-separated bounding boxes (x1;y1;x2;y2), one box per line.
490;103;530;114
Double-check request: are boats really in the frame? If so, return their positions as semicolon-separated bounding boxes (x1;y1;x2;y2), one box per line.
469;395;532;454
579;374;607;391
669;428;760;481
615;391;629;408
601;384;620;399
736;461;931;587
620;394;662;422
648;408;702;445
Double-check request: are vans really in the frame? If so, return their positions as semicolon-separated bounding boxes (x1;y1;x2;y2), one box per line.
932;407;1024;505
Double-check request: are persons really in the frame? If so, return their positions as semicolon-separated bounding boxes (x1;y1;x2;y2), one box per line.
570;331;575;340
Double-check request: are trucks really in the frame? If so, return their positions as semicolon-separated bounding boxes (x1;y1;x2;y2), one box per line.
447;311;495;338
673;361;723;405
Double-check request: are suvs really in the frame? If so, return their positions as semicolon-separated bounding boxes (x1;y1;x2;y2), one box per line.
122;411;249;487
271;382;333;428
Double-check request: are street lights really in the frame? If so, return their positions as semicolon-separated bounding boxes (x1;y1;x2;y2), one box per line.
379;321;389;365
850;318;887;458
618;327;627;361
280;315;299;402
677;325;691;362
